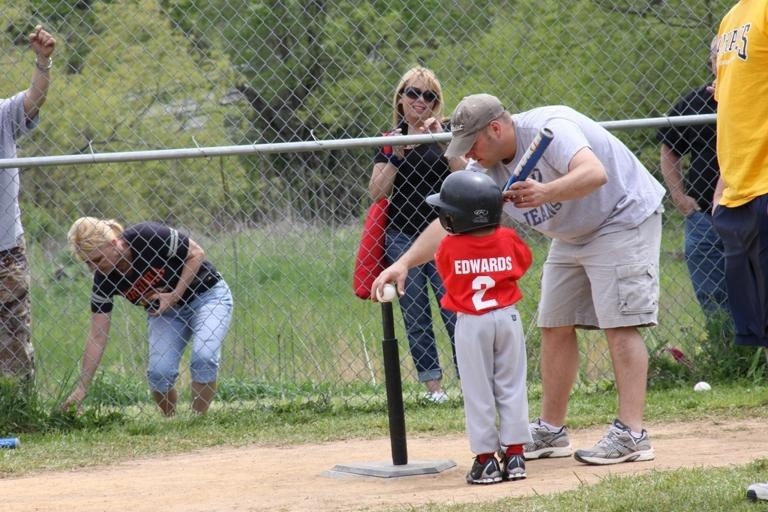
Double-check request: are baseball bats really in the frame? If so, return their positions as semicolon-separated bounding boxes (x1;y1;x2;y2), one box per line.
500;128;553;198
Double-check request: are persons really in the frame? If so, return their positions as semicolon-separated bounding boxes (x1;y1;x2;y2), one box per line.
367;67;469;404
713;1;768;502
656;31;737;354
424;169;534;484
51;216;234;418
0;25;56;408
371;91;667;465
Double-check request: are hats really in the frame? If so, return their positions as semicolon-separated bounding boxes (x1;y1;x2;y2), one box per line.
444;93;505;158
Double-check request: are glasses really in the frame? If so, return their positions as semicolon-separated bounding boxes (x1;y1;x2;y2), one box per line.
400;85;437;103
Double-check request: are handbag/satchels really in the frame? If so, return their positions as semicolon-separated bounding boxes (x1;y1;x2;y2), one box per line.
355;199;388;297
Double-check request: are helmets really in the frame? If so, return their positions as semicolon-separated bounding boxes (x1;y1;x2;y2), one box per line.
425;170;503;235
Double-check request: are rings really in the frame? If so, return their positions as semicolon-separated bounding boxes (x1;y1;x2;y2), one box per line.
520;195;525;202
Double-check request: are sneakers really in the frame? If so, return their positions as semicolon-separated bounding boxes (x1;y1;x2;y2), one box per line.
501;417;572;460
574;419;655;465
466;455;502;483
499;452;527;480
745;482;767;499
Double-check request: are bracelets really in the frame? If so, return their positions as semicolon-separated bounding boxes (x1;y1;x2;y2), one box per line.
34;57;52;71
387;152;404;169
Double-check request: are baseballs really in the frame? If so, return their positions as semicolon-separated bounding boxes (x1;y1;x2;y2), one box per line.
376;285;395;303
694;380;710;392
